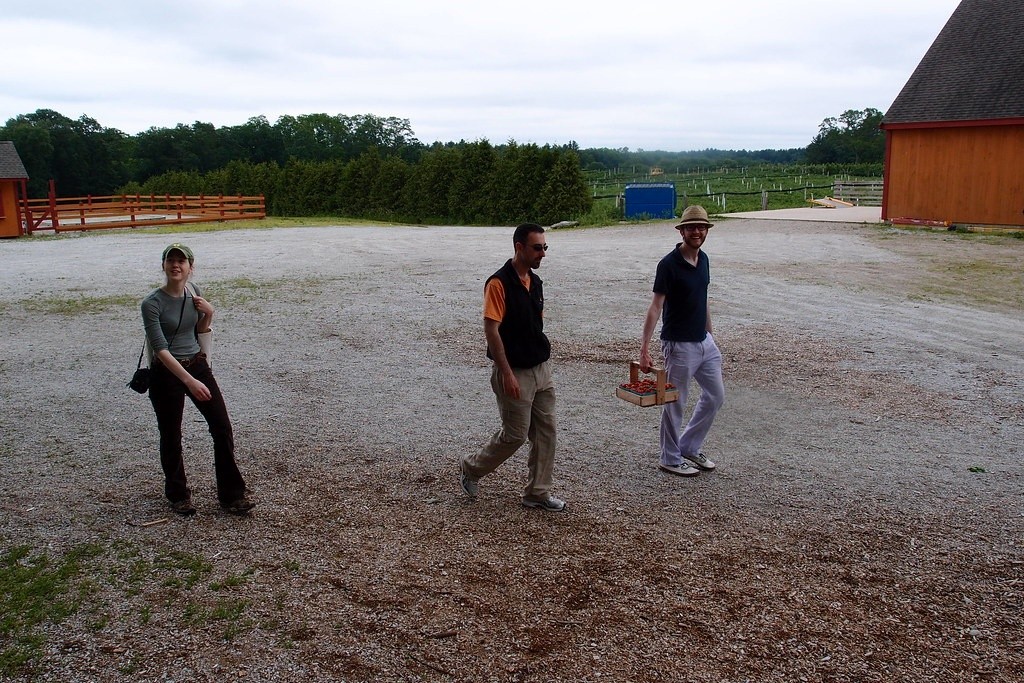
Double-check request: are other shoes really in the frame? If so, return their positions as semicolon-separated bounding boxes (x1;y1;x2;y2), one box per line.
171;500;196;515
220;498;256;513
659;452;717;476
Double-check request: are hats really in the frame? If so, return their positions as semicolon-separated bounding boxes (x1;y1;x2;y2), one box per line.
674;205;714;230
162;243;194;259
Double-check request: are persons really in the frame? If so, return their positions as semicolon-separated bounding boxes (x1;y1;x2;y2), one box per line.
141;242;255;515
459;223;567;511
638;204;726;477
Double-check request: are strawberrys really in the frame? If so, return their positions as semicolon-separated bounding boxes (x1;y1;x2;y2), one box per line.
620;378;676;393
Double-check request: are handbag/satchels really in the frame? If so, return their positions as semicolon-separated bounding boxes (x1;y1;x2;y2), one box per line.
126;368;152;393
197;327;212;371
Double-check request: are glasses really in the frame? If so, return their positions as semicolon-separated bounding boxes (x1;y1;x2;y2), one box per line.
525;243;549;251
683;225;706;231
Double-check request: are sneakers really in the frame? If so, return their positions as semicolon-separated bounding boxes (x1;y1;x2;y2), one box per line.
458;455;478;498
522;495;566;511
